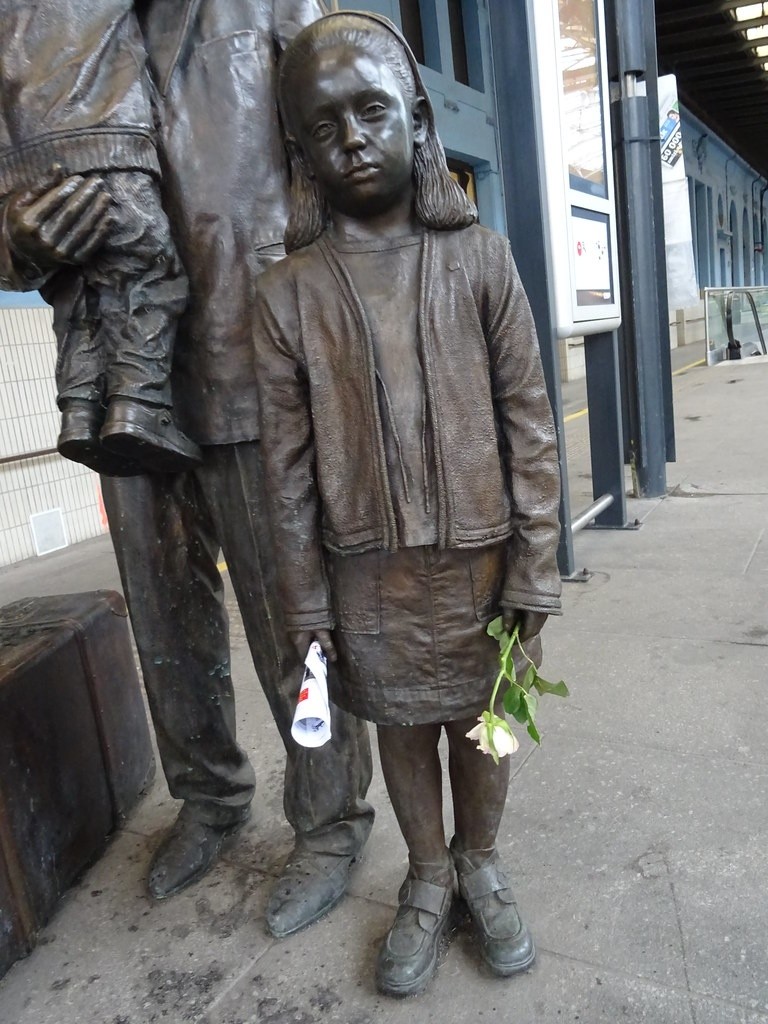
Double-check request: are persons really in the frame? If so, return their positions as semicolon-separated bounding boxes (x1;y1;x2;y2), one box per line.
0;0;563;997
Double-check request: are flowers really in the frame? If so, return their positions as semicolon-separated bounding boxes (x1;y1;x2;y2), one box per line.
465;614;568;764
